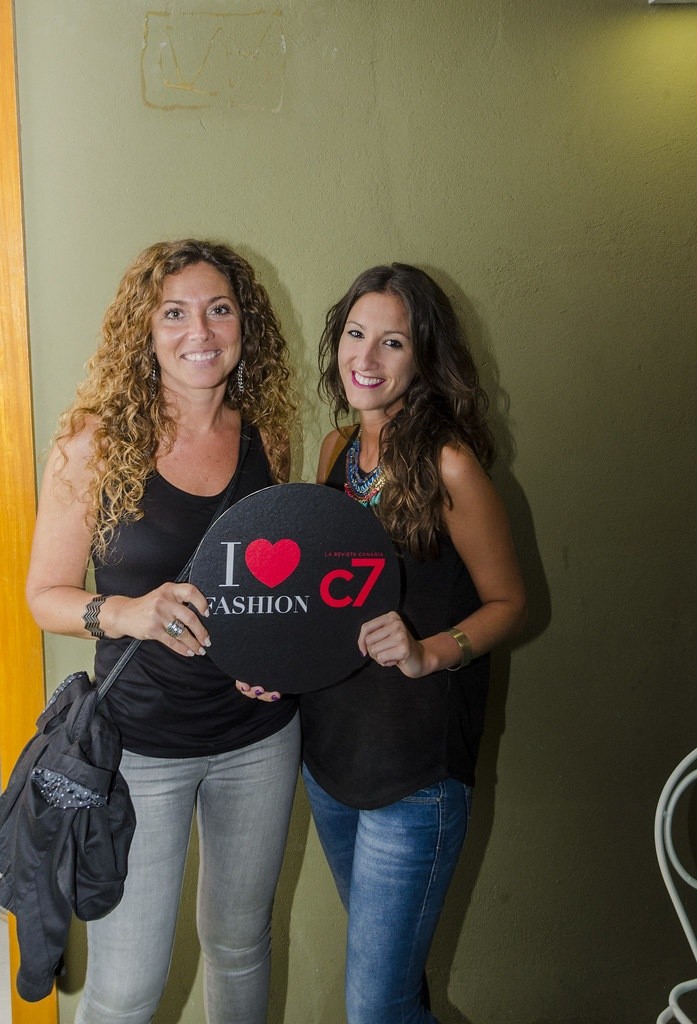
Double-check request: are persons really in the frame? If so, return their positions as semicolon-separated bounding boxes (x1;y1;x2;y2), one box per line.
26;237;528;1024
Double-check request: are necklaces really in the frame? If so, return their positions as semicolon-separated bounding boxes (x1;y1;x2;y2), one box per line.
345;428;394;508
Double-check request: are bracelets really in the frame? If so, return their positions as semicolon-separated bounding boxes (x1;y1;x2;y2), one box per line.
442;627;472;673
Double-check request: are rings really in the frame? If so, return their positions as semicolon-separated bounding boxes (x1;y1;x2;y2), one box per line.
165;618;185;638
